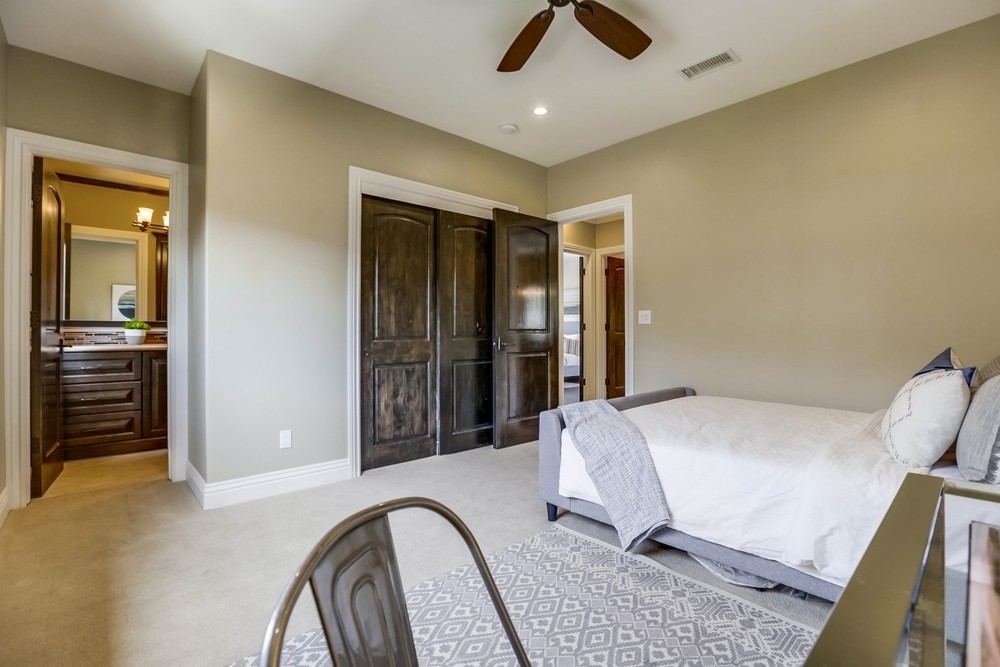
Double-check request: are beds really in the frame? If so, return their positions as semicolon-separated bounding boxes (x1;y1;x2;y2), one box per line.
540;387;1000;632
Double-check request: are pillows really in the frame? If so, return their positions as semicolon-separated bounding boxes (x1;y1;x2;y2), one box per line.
980;356;1000;382
961;366;984;391
881;347;971;476
955;374;1000;481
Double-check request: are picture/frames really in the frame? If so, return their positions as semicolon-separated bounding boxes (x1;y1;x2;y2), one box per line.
112;284;136;321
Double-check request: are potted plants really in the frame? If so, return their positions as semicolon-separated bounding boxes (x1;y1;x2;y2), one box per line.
123;318;151;344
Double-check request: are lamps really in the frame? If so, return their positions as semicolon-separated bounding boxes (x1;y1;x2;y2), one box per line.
133;207;169;233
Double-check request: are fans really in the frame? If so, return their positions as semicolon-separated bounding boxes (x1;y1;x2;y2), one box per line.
496;0;652;72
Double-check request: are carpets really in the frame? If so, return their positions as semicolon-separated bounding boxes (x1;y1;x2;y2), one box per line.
227;524;822;667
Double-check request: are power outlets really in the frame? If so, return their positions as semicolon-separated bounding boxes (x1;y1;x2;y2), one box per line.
280;430;292;449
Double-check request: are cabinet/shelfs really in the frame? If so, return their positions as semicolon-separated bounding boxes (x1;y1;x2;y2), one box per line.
61;351;168;461
151;231;168;321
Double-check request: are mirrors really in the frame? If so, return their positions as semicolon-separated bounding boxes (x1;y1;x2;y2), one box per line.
64;223;149;321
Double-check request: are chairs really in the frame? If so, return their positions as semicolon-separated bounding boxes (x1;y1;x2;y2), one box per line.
261;497;532;667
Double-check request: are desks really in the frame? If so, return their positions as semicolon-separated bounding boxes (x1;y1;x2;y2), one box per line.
803;473;1000;667
